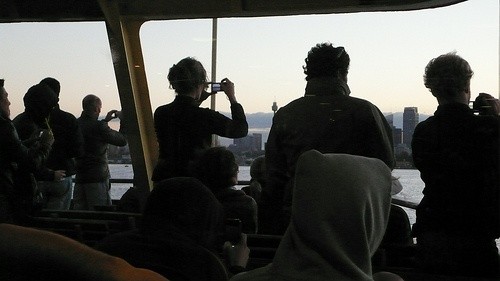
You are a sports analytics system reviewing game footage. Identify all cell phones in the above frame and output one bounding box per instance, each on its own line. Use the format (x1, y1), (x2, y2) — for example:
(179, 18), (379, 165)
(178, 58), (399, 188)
(224, 219), (242, 246)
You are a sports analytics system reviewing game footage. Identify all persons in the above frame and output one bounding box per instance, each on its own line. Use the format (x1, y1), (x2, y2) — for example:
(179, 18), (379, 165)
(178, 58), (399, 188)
(410, 51), (500, 281)
(263, 43), (395, 235)
(151, 58), (248, 188)
(0, 75), (404, 281)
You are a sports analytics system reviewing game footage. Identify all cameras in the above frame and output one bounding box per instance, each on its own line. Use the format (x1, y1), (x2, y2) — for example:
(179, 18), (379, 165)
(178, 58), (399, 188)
(115, 111), (122, 117)
(468, 101), (478, 112)
(212, 83), (224, 91)
